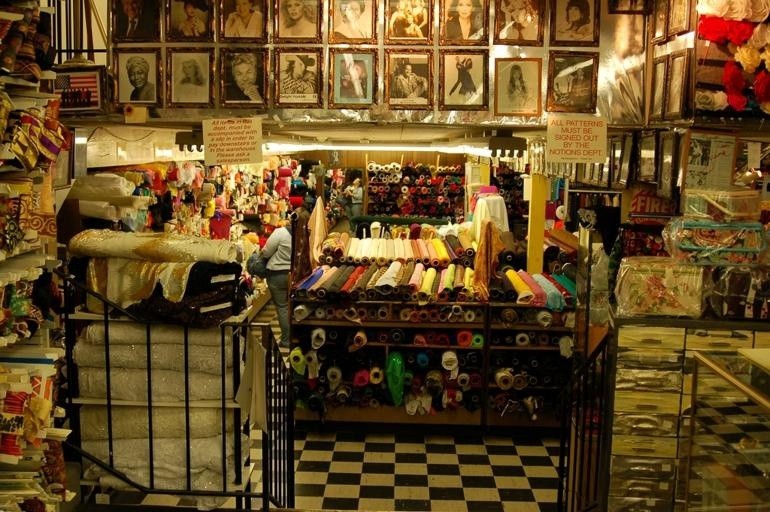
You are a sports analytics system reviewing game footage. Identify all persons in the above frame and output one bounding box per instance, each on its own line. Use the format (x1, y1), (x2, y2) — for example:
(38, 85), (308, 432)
(690, 135), (707, 165)
(125, 46), (590, 114)
(259, 202), (306, 347)
(108, 1), (592, 46)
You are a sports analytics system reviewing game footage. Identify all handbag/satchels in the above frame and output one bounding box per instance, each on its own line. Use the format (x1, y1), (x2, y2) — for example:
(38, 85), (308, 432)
(247, 253), (267, 279)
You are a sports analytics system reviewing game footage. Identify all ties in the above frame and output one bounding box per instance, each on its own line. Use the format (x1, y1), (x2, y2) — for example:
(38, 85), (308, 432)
(127, 19), (136, 36)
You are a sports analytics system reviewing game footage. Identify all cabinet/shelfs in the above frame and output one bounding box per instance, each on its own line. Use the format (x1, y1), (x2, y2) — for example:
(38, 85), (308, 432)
(284, 282), (613, 428)
(60, 258), (249, 512)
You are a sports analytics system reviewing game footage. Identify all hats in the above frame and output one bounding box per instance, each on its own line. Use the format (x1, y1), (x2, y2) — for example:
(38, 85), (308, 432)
(286, 55), (315, 66)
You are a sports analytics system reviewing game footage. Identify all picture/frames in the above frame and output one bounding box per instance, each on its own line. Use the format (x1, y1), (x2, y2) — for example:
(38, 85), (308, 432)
(546, 50), (600, 114)
(438, 48), (489, 111)
(549, 1), (600, 47)
(112, 47), (163, 109)
(383, 47), (434, 110)
(219, 47), (269, 110)
(166, 47), (217, 108)
(328, 47), (380, 110)
(439, 1), (489, 46)
(216, 1), (268, 44)
(51, 65), (107, 115)
(328, 2), (378, 45)
(493, 1), (546, 47)
(164, 2), (215, 43)
(52, 128), (74, 191)
(110, 1), (162, 43)
(273, 46), (323, 110)
(384, 2), (434, 45)
(581, 0), (691, 202)
(272, 1), (323, 43)
(494, 58), (542, 117)
